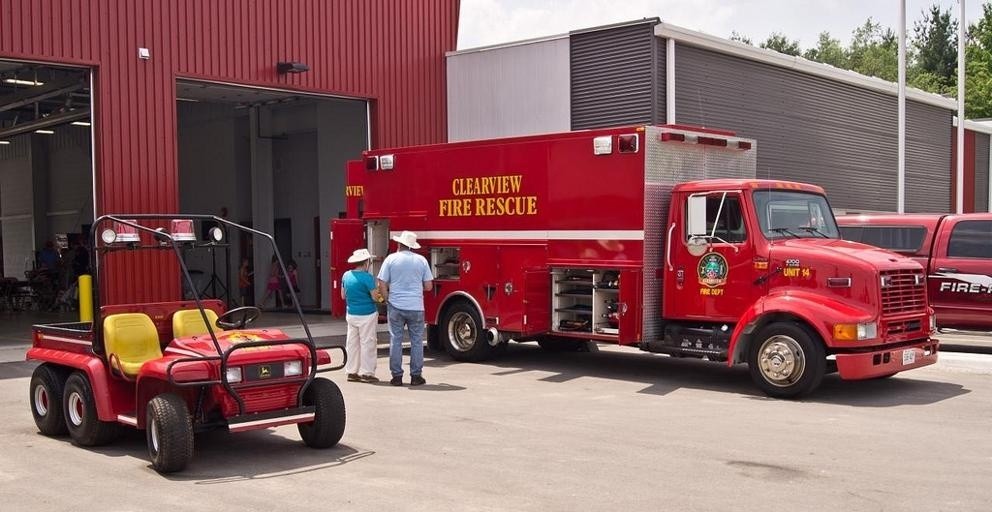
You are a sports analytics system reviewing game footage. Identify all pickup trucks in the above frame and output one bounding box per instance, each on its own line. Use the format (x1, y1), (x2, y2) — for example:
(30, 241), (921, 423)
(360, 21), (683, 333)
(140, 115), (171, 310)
(805, 211), (992, 340)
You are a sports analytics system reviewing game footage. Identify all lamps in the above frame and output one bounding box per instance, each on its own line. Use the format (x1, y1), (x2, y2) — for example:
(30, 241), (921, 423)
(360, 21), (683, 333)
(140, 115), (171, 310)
(278, 61), (310, 73)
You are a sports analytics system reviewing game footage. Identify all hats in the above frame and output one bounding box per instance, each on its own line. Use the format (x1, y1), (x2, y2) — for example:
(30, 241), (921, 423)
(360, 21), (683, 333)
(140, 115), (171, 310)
(391, 230), (422, 251)
(347, 248), (379, 264)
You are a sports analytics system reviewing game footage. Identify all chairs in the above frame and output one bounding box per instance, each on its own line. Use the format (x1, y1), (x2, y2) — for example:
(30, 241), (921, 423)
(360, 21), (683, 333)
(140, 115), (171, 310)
(171, 307), (226, 341)
(103, 314), (164, 376)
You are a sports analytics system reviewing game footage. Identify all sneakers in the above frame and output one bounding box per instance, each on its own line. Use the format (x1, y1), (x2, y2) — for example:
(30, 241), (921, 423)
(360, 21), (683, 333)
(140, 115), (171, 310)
(346, 373), (360, 382)
(390, 375), (403, 385)
(411, 374), (426, 385)
(361, 374), (379, 383)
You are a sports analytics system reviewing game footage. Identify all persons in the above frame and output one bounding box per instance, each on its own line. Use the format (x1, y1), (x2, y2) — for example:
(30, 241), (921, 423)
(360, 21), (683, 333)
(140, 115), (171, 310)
(282, 260), (298, 301)
(259, 254), (288, 309)
(340, 248), (381, 383)
(377, 231), (434, 386)
(239, 257), (253, 306)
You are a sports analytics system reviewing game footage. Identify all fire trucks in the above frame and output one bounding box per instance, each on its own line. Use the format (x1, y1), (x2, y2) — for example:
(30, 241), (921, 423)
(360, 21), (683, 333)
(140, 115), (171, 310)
(327, 116), (940, 403)
